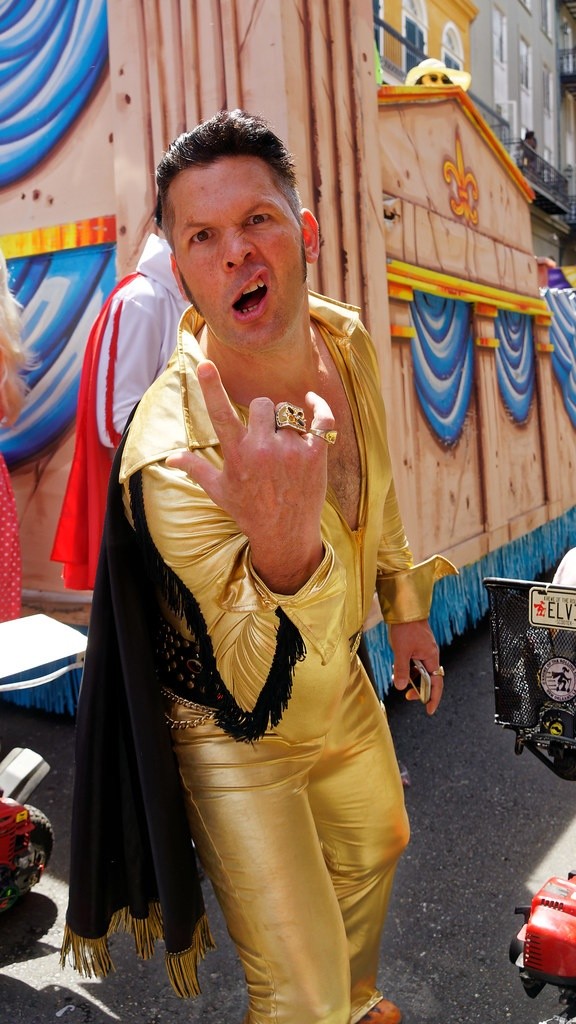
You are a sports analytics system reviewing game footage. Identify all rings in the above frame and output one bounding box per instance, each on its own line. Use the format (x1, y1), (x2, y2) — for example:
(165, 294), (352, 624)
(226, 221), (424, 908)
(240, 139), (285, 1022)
(430, 666), (444, 676)
(310, 428), (337, 444)
(276, 402), (307, 434)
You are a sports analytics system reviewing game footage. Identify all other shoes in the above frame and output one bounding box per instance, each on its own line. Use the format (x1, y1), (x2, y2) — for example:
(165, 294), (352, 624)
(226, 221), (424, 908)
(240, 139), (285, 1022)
(348, 986), (402, 1024)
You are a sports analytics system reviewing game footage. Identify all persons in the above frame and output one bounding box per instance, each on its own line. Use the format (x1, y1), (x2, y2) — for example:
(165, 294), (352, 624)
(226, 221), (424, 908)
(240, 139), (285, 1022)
(57, 110), (457, 1023)
(0, 250), (39, 621)
(53, 192), (190, 592)
